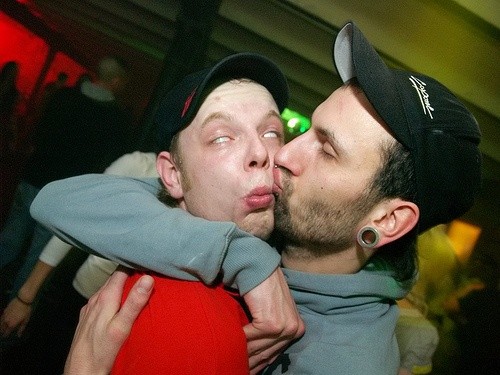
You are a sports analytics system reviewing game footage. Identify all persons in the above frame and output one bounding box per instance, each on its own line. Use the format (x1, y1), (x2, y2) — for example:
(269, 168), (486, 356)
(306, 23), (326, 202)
(0, 56), (146, 305)
(394, 228), (468, 374)
(0, 151), (160, 375)
(104, 52), (289, 374)
(27, 17), (482, 375)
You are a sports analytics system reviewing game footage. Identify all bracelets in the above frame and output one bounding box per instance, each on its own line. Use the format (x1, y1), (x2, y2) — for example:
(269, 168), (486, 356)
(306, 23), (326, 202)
(15, 294), (35, 306)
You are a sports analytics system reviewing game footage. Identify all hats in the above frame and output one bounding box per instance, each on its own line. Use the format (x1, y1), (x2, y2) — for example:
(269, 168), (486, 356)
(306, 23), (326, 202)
(331, 23), (485, 216)
(151, 50), (291, 154)
